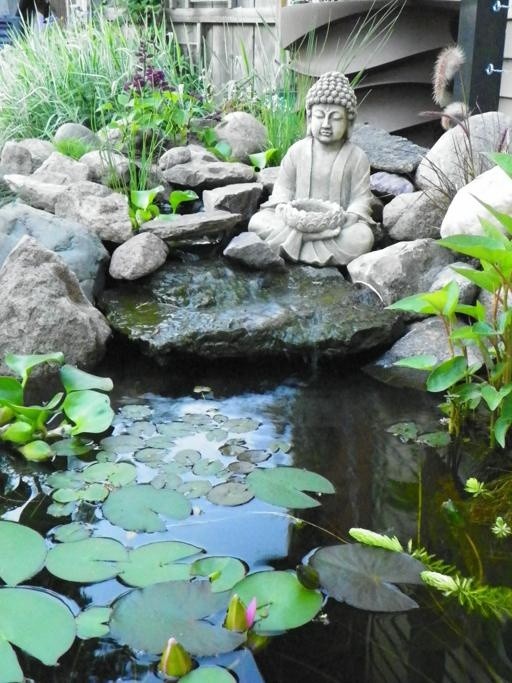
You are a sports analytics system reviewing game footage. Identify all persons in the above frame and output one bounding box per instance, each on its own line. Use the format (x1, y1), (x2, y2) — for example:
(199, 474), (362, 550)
(245, 70), (378, 268)
